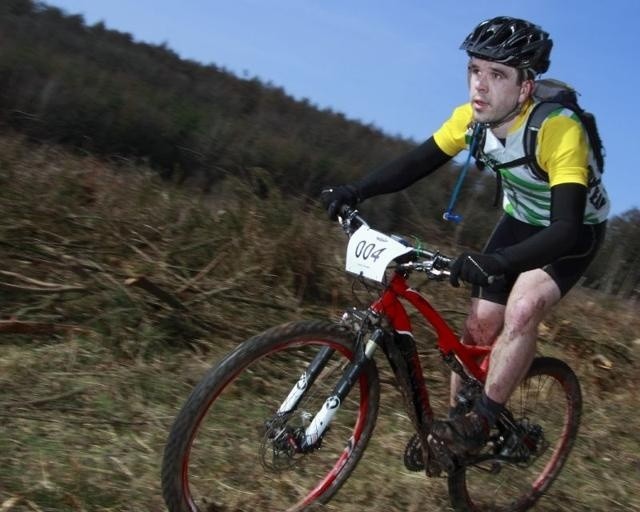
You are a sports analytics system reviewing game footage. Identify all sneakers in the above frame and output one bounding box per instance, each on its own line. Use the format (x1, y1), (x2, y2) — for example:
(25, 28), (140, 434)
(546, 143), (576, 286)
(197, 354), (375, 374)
(432, 412), (487, 460)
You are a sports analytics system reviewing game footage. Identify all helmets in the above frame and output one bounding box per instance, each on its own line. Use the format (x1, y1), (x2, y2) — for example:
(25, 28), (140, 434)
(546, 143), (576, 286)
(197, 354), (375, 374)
(460, 16), (552, 80)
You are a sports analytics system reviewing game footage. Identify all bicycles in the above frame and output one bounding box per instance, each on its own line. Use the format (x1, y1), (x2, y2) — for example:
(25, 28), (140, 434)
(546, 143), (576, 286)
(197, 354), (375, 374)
(162, 185), (583, 512)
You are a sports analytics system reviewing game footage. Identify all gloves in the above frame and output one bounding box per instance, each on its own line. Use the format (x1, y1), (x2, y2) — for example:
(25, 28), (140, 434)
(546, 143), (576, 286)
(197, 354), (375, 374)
(450, 253), (504, 287)
(320, 184), (357, 221)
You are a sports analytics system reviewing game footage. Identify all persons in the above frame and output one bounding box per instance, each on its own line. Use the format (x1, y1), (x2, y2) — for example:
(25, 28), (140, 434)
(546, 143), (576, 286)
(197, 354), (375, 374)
(321, 17), (612, 455)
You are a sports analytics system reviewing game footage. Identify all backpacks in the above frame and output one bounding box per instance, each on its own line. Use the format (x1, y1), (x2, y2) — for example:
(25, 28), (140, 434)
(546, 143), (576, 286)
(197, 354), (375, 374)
(530, 78), (603, 187)
